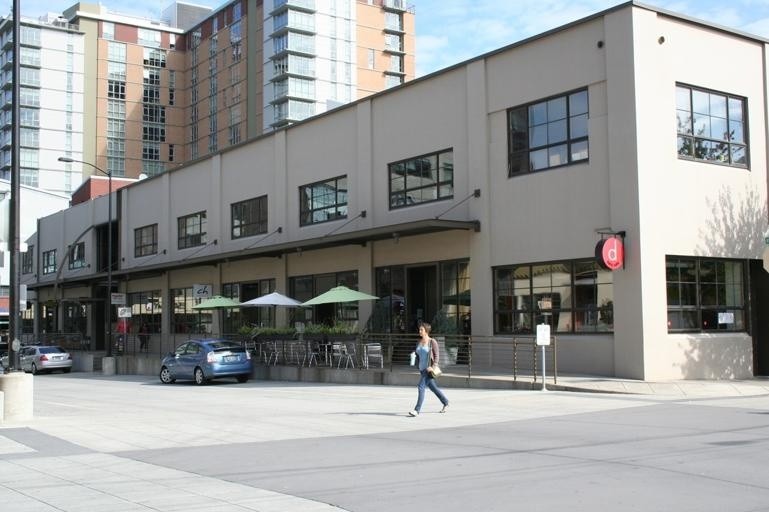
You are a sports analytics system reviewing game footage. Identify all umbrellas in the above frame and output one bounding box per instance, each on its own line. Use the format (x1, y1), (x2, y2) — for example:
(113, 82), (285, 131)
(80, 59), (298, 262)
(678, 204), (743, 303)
(299, 286), (382, 322)
(237, 292), (313, 329)
(442, 289), (470, 307)
(191, 296), (244, 339)
(378, 294), (405, 307)
(340, 303), (358, 311)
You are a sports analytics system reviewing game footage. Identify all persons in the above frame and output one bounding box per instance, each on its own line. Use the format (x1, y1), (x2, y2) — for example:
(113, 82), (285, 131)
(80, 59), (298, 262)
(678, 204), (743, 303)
(115, 317), (134, 356)
(462, 309), (471, 356)
(137, 322), (152, 354)
(408, 323), (451, 417)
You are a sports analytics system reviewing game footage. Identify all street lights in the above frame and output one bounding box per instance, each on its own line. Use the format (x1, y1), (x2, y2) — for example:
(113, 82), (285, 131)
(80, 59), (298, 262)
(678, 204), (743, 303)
(56, 153), (118, 379)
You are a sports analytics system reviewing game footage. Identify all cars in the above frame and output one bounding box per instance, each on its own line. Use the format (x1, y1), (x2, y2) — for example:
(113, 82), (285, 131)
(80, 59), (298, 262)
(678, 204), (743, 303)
(158, 338), (256, 387)
(0, 342), (77, 378)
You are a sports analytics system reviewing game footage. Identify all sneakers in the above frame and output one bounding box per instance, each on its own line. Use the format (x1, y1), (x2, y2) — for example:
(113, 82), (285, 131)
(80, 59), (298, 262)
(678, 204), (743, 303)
(440, 403), (450, 413)
(409, 410), (419, 416)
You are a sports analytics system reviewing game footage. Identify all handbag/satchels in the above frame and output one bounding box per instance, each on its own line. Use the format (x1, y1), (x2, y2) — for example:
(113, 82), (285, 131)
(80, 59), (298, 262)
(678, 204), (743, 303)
(430, 365), (442, 379)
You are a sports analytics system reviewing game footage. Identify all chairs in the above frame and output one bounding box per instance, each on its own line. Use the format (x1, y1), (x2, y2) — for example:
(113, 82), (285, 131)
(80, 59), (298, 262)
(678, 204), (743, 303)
(244, 339), (384, 370)
